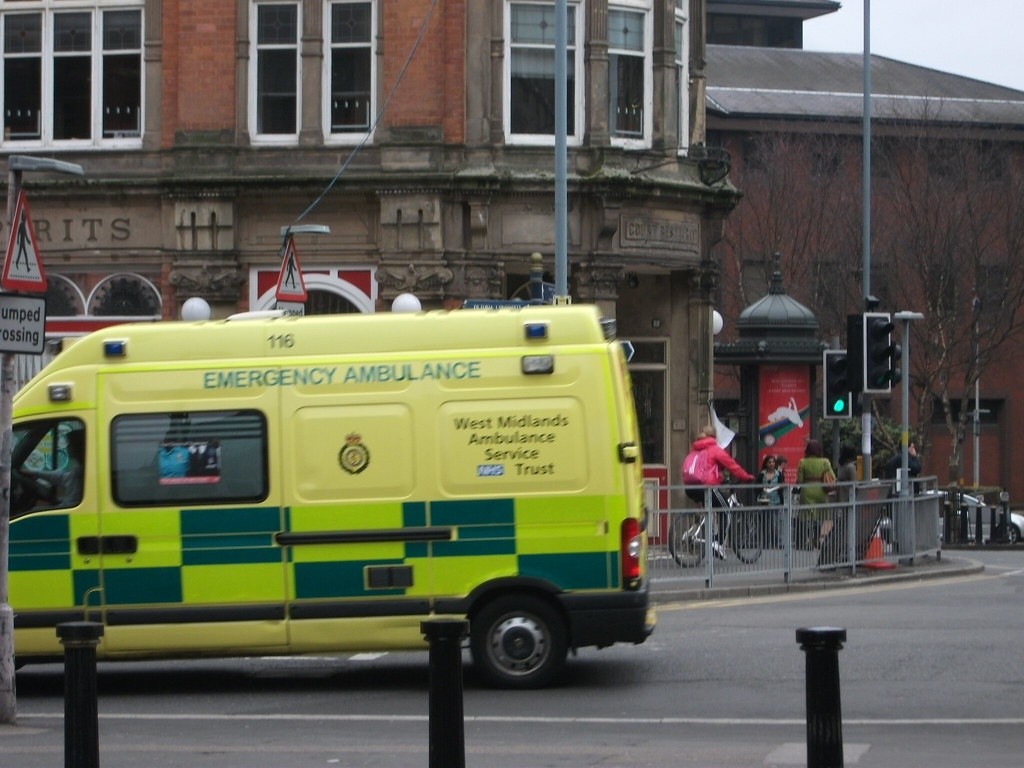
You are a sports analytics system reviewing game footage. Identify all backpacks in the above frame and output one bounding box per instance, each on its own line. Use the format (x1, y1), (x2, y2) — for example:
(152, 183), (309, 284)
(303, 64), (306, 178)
(682, 449), (708, 484)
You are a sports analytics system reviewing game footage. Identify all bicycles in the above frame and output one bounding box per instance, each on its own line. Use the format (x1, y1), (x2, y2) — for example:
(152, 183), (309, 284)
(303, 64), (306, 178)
(668, 478), (764, 569)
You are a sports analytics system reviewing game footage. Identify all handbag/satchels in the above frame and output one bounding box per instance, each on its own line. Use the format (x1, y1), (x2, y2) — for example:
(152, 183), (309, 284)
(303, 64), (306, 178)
(823, 471), (834, 488)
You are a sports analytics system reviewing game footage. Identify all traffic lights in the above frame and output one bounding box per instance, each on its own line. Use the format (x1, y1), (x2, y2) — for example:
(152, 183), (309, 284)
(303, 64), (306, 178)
(864, 313), (894, 393)
(823, 351), (852, 419)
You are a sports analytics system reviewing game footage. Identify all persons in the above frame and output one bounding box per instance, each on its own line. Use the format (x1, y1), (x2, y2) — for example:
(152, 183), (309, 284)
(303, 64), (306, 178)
(775, 456), (787, 474)
(838, 445), (860, 511)
(884, 438), (922, 553)
(681, 424), (756, 560)
(795, 440), (837, 550)
(19, 428), (86, 509)
(756, 454), (788, 546)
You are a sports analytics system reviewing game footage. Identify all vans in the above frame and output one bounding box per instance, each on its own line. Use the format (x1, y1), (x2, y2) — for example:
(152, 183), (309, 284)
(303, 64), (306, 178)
(10, 303), (659, 691)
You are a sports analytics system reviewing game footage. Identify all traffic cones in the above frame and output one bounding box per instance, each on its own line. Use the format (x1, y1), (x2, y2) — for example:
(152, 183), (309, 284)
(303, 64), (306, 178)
(862, 525), (897, 569)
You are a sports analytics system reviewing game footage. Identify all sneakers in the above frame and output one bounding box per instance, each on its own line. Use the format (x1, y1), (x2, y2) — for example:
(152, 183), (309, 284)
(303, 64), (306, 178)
(713, 541), (729, 560)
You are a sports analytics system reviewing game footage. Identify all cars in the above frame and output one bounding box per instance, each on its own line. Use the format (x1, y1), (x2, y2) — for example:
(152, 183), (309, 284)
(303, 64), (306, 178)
(879, 490), (1024, 545)
